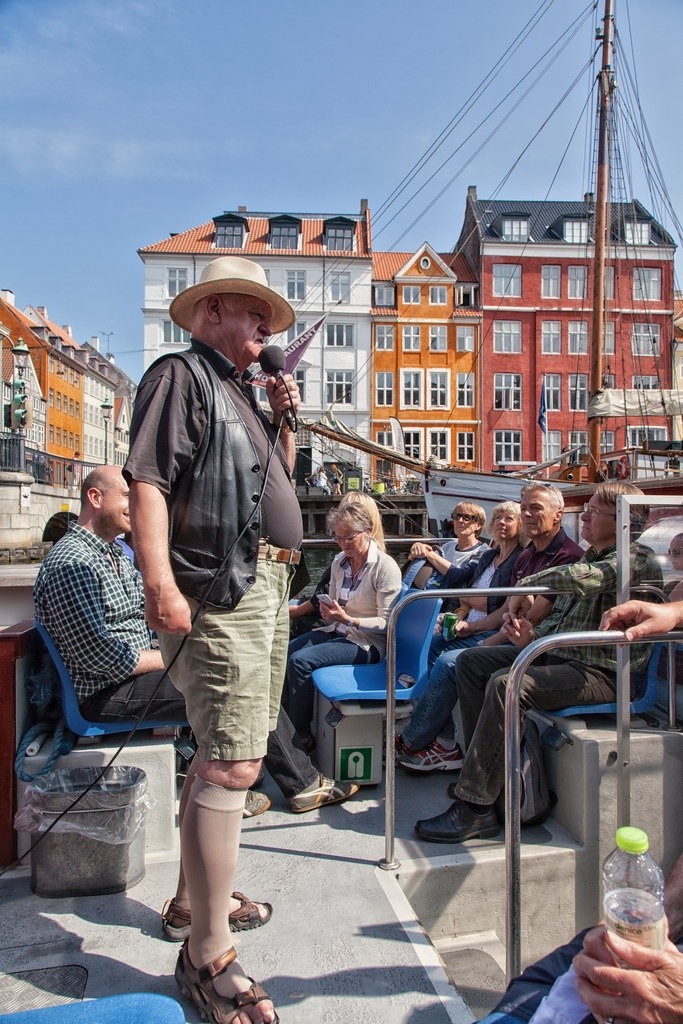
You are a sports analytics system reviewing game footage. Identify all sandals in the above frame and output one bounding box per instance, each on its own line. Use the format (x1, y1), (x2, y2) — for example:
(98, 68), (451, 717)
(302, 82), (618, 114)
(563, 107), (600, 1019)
(161, 892), (273, 942)
(175, 936), (279, 1024)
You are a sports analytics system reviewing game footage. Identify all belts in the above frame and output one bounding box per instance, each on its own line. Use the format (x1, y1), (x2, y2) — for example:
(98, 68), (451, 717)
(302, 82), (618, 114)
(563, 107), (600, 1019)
(257, 546), (302, 566)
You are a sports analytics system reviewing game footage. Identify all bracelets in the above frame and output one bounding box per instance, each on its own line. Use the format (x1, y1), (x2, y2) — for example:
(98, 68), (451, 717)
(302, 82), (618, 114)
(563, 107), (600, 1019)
(454, 608), (467, 615)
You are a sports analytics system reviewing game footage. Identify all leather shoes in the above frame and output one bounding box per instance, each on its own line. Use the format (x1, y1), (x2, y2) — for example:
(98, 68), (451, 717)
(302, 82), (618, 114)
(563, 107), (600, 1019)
(448, 782), (457, 797)
(415, 799), (499, 843)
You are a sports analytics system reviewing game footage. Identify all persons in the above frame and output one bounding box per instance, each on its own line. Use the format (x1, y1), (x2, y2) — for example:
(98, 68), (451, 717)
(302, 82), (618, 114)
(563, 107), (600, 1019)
(32, 442), (53, 485)
(472, 852), (683, 1024)
(657, 532), (683, 683)
(385, 469), (395, 495)
(33, 464), (404, 817)
(70, 451), (81, 490)
(598, 600), (683, 647)
(413, 480), (663, 843)
(407, 501), (531, 623)
(123, 256), (302, 1024)
(382, 482), (586, 771)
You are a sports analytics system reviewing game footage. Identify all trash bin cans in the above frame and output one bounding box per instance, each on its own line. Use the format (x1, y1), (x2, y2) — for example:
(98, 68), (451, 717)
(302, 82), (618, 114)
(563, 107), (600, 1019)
(26, 765), (148, 898)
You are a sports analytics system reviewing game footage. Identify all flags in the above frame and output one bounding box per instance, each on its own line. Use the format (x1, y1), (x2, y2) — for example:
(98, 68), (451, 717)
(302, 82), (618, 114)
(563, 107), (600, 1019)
(538, 381), (547, 433)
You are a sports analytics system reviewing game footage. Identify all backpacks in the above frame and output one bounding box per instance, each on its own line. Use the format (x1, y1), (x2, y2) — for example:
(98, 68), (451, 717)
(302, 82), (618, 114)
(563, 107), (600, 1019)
(400, 545), (450, 600)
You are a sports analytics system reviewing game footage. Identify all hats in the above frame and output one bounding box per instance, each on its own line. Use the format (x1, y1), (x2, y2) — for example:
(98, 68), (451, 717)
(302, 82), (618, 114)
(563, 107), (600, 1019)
(169, 256), (296, 335)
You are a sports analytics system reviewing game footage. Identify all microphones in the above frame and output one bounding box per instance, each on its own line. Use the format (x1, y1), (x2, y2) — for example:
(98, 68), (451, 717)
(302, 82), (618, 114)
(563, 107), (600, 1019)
(259, 345), (296, 434)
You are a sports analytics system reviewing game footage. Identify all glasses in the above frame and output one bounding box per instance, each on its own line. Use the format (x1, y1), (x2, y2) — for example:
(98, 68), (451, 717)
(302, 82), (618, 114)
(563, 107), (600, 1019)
(584, 503), (614, 517)
(543, 484), (560, 503)
(334, 533), (358, 543)
(450, 513), (476, 521)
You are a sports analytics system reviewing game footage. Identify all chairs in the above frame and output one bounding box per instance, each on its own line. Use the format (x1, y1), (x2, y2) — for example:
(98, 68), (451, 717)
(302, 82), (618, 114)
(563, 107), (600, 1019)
(312, 540), (667, 714)
(37, 623), (195, 740)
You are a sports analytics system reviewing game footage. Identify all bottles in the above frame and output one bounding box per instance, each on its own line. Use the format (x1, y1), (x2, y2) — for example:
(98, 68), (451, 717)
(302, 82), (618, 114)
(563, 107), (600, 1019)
(602, 826), (668, 969)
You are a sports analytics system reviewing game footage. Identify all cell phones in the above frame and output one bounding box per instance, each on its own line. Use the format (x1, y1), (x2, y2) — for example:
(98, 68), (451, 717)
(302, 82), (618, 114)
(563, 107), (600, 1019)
(317, 594), (335, 610)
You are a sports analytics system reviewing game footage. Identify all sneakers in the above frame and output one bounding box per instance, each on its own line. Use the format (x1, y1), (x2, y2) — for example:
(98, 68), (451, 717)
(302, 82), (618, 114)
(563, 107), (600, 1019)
(381, 734), (465, 770)
(290, 773), (359, 812)
(242, 790), (272, 817)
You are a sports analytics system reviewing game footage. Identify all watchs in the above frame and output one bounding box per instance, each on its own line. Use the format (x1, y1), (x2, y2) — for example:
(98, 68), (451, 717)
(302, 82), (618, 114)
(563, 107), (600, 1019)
(477, 640), (484, 646)
(347, 618), (354, 627)
